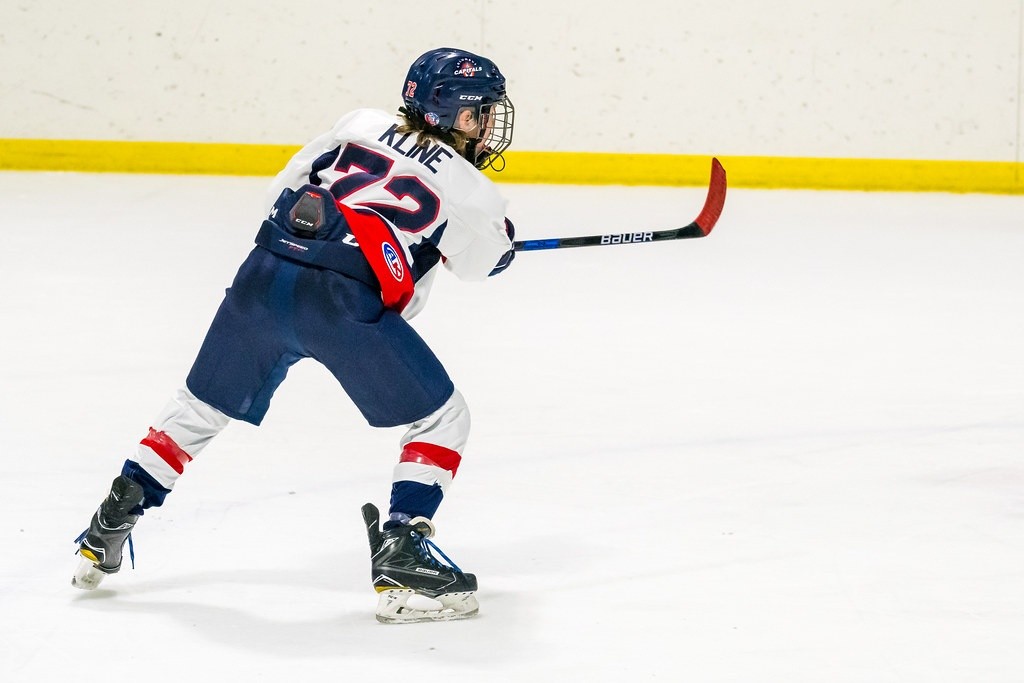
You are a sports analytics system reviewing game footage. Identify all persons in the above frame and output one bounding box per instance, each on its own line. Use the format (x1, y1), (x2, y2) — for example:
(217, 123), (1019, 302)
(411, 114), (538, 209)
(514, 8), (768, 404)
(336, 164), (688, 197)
(70, 46), (516, 624)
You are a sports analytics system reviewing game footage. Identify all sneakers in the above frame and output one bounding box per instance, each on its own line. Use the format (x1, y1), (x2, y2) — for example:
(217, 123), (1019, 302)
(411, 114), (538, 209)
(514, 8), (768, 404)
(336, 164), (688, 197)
(361, 502), (480, 624)
(72, 476), (144, 590)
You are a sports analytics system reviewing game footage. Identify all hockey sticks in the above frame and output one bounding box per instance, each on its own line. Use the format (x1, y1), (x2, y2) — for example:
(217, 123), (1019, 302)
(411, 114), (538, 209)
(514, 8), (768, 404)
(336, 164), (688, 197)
(513, 155), (727, 252)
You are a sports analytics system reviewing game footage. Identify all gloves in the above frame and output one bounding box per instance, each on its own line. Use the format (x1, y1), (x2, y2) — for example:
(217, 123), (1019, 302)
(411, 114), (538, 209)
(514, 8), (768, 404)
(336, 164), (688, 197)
(488, 216), (515, 276)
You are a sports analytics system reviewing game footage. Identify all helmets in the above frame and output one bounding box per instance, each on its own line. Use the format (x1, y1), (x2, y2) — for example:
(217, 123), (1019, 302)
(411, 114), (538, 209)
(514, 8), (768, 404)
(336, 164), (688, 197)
(401, 48), (514, 170)
